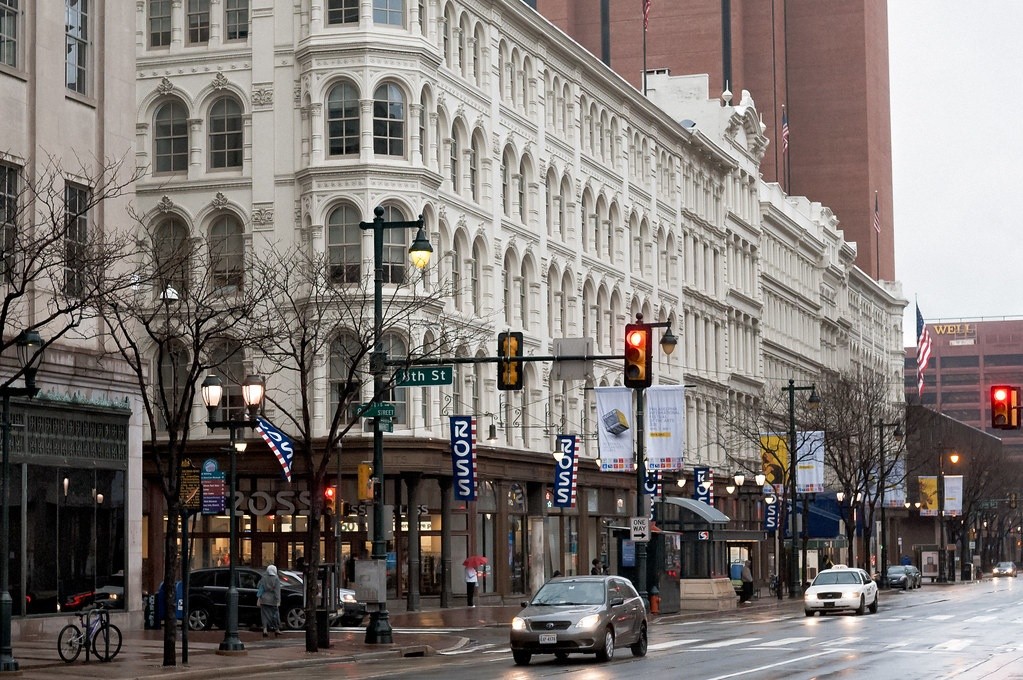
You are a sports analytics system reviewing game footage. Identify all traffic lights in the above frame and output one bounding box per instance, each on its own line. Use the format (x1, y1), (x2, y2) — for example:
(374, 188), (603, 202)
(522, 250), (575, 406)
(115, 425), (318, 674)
(623, 324), (652, 389)
(355, 462), (374, 503)
(990, 384), (1012, 430)
(325, 487), (336, 516)
(341, 499), (350, 518)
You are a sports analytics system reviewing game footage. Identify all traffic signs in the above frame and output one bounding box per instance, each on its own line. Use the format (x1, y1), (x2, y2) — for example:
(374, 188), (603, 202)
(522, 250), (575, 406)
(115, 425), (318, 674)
(629, 516), (651, 542)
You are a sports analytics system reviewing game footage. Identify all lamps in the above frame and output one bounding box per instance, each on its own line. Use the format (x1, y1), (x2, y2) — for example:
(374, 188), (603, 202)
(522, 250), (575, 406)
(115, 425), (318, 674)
(487, 414), (498, 443)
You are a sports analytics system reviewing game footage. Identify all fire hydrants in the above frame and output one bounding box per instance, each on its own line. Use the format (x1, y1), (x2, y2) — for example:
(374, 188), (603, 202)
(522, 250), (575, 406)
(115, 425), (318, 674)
(649, 586), (661, 615)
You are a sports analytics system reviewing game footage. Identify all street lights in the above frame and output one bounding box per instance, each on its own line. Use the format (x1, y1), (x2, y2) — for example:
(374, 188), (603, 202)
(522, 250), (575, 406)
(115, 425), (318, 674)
(782, 380), (820, 600)
(63, 477), (104, 593)
(634, 312), (678, 615)
(202, 374), (264, 657)
(874, 417), (905, 590)
(360, 205), (434, 643)
(0, 329), (45, 671)
(932, 442), (960, 582)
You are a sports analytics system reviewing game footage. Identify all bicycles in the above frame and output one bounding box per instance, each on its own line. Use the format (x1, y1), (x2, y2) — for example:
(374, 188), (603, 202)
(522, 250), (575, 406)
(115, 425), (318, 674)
(57, 600), (122, 664)
(769, 574), (778, 597)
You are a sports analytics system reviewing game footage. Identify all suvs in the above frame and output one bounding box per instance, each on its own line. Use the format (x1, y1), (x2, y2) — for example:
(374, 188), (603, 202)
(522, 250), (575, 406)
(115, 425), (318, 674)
(510, 575), (648, 664)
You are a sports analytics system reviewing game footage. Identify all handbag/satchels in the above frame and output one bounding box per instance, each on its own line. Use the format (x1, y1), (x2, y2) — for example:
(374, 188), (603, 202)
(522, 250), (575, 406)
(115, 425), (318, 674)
(256, 577), (264, 597)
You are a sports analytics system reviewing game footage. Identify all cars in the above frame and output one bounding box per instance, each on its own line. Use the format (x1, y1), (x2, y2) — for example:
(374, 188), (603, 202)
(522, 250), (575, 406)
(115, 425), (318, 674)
(185, 566), (356, 629)
(887, 565), (922, 590)
(66, 571), (125, 610)
(804, 565), (880, 617)
(993, 561), (1017, 578)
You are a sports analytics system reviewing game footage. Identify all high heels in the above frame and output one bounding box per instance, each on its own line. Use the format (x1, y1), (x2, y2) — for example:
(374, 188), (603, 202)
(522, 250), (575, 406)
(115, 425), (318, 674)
(263, 633), (268, 637)
(275, 632), (284, 637)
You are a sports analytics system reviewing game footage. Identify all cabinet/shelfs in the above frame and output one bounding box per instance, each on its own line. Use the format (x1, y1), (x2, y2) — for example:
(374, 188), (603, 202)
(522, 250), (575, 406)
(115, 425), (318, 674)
(421, 556), (432, 594)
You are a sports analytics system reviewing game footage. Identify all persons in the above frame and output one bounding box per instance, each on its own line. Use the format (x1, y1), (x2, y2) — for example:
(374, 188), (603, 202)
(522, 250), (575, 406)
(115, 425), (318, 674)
(591, 559), (609, 575)
(741, 559), (753, 604)
(901, 554), (911, 565)
(822, 555), (834, 569)
(255, 564), (286, 638)
(464, 566), (480, 608)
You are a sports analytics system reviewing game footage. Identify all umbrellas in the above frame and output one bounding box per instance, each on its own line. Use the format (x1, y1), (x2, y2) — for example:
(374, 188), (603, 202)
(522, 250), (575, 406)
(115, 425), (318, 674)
(462, 554), (488, 568)
